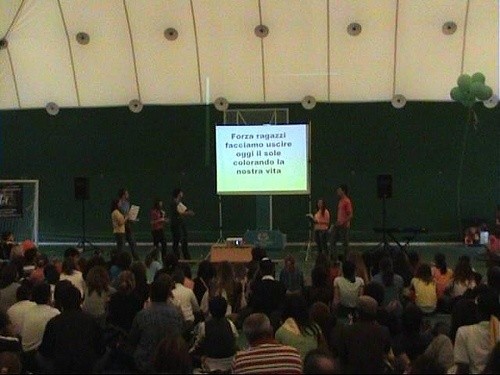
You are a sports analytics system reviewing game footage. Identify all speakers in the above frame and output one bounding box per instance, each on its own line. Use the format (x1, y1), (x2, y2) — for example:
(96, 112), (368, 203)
(377, 174), (392, 198)
(74, 177), (90, 201)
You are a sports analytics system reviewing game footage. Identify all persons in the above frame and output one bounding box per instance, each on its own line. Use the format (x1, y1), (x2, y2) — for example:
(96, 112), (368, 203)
(119, 189), (141, 261)
(111, 197), (131, 253)
(312, 199), (330, 255)
(0, 215), (500, 375)
(170, 189), (193, 260)
(149, 199), (167, 259)
(329, 185), (352, 258)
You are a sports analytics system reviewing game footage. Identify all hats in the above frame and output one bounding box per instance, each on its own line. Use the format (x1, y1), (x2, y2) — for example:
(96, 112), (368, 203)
(261, 258), (273, 270)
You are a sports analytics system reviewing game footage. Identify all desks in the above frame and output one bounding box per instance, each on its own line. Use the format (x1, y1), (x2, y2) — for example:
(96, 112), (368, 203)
(374, 227), (429, 251)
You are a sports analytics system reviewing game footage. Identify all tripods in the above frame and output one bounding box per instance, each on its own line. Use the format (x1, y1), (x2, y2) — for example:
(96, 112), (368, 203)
(76, 200), (95, 254)
(373, 198), (395, 253)
